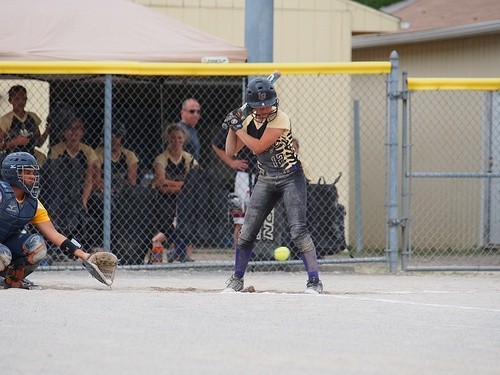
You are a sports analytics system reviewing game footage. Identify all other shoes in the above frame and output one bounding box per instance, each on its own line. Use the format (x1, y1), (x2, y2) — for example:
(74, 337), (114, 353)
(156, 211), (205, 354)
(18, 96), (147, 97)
(221, 275), (244, 293)
(304, 276), (324, 295)
(0, 274), (42, 291)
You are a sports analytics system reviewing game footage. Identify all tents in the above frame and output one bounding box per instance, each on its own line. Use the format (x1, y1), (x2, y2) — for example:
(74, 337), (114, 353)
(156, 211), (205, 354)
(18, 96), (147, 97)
(0, 0), (246, 246)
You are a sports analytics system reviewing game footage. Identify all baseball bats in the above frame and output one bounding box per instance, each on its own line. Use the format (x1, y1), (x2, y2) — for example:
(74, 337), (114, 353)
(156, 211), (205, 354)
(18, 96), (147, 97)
(221, 71), (282, 129)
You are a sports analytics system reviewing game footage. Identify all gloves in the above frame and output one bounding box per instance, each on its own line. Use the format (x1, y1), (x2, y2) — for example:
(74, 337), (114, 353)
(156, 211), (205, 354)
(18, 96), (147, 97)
(224, 109), (245, 132)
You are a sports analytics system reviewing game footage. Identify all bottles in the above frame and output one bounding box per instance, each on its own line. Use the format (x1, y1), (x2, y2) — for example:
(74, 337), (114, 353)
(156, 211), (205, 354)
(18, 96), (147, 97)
(19, 125), (28, 136)
(144, 171), (154, 186)
(153, 240), (163, 264)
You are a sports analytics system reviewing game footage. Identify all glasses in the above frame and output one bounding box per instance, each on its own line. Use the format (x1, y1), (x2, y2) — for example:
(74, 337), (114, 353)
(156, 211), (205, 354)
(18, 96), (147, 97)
(183, 109), (201, 114)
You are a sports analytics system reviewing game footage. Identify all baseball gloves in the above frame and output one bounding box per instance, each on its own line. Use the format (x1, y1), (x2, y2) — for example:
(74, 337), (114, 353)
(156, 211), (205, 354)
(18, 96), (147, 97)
(84, 252), (118, 287)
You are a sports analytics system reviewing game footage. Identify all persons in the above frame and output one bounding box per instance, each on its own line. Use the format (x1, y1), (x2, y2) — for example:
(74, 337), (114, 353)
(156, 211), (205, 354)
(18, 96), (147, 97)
(225, 77), (323, 294)
(177, 98), (200, 260)
(0, 152), (118, 289)
(230, 136), (259, 243)
(293, 138), (298, 154)
(0, 85), (50, 158)
(95, 128), (139, 191)
(154, 123), (201, 194)
(50, 118), (98, 212)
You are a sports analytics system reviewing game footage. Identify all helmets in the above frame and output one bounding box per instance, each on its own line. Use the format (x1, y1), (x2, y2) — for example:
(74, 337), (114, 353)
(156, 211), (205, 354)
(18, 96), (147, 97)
(245, 76), (278, 109)
(1, 151), (41, 188)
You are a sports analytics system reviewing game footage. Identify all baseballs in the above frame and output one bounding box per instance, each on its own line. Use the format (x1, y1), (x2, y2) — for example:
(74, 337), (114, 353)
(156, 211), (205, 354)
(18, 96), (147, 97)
(274, 246), (290, 261)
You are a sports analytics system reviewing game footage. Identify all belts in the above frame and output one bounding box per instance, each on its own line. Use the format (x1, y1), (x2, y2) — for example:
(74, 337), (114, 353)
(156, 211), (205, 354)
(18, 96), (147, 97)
(259, 161), (302, 177)
